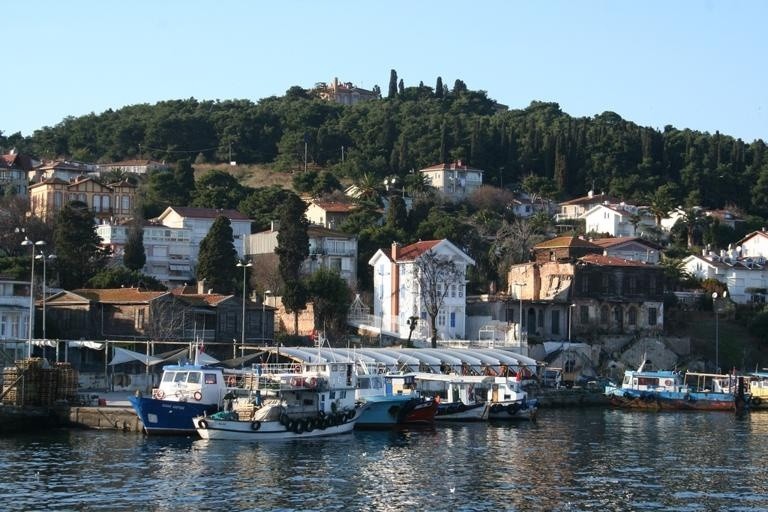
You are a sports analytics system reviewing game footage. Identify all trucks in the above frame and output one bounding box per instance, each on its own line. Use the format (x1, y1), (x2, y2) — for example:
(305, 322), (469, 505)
(540, 367), (575, 390)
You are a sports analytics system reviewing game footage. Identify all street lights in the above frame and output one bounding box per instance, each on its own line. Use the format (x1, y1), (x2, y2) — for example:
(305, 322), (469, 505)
(235, 259), (254, 370)
(568, 304), (576, 376)
(262, 290), (271, 347)
(514, 283), (527, 354)
(20, 236), (50, 369)
(712, 290), (727, 368)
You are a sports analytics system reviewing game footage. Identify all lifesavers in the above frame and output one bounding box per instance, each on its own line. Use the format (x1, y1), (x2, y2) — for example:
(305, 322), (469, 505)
(290, 378), (296, 386)
(194, 392), (202, 400)
(665, 380), (670, 386)
(154, 390), (164, 399)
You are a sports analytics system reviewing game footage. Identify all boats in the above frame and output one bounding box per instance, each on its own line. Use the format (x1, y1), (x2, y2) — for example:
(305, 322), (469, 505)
(601, 352), (768, 411)
(190, 358), (372, 439)
(0, 405), (51, 435)
(127, 335), (539, 439)
